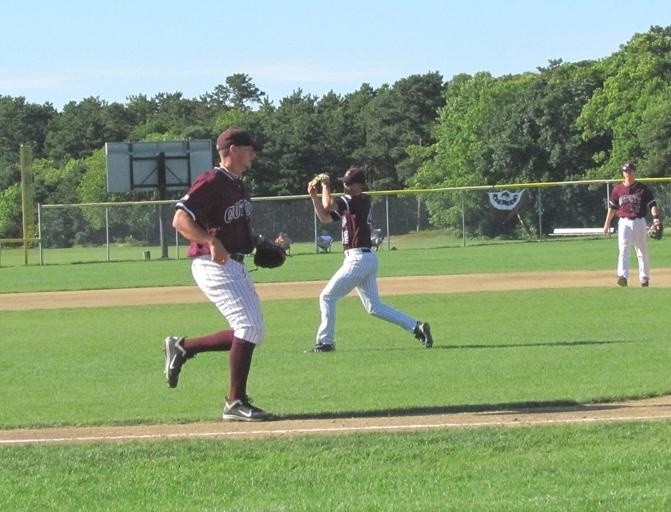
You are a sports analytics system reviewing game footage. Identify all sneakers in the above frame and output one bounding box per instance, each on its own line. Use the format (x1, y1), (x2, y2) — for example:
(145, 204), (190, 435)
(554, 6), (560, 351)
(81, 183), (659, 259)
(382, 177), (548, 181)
(163, 336), (187, 388)
(303, 342), (336, 354)
(639, 278), (649, 287)
(617, 276), (627, 287)
(222, 395), (274, 421)
(413, 321), (433, 349)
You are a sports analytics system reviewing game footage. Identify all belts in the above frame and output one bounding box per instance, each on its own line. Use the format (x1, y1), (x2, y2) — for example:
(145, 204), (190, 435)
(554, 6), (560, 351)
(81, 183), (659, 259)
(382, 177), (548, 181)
(345, 249), (371, 256)
(620, 216), (643, 220)
(230, 254), (244, 262)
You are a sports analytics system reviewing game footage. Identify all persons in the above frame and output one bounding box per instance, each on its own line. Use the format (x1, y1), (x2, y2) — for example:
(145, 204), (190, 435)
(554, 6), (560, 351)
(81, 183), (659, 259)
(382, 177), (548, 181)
(303, 169), (433, 353)
(602, 163), (663, 286)
(275, 232), (290, 249)
(371, 228), (383, 250)
(318, 229), (332, 252)
(166, 128), (273, 422)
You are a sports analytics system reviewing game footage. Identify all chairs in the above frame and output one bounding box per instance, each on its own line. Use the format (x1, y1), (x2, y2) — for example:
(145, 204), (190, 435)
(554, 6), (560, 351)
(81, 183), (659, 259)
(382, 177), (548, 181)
(317, 235), (334, 253)
(280, 238), (292, 256)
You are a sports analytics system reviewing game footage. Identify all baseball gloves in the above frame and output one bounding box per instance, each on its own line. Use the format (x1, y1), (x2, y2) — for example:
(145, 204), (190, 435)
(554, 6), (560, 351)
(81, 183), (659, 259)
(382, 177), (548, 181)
(254, 240), (285, 268)
(647, 223), (662, 240)
(311, 173), (329, 186)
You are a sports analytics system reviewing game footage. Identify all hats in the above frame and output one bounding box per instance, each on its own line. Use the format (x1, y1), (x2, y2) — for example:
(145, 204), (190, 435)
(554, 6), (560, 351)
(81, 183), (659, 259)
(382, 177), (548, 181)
(343, 169), (367, 184)
(216, 128), (262, 152)
(622, 163), (637, 172)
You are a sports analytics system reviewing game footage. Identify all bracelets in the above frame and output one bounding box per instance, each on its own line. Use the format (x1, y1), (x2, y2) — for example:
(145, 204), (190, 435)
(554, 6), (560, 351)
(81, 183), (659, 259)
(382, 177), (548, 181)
(652, 215), (660, 219)
